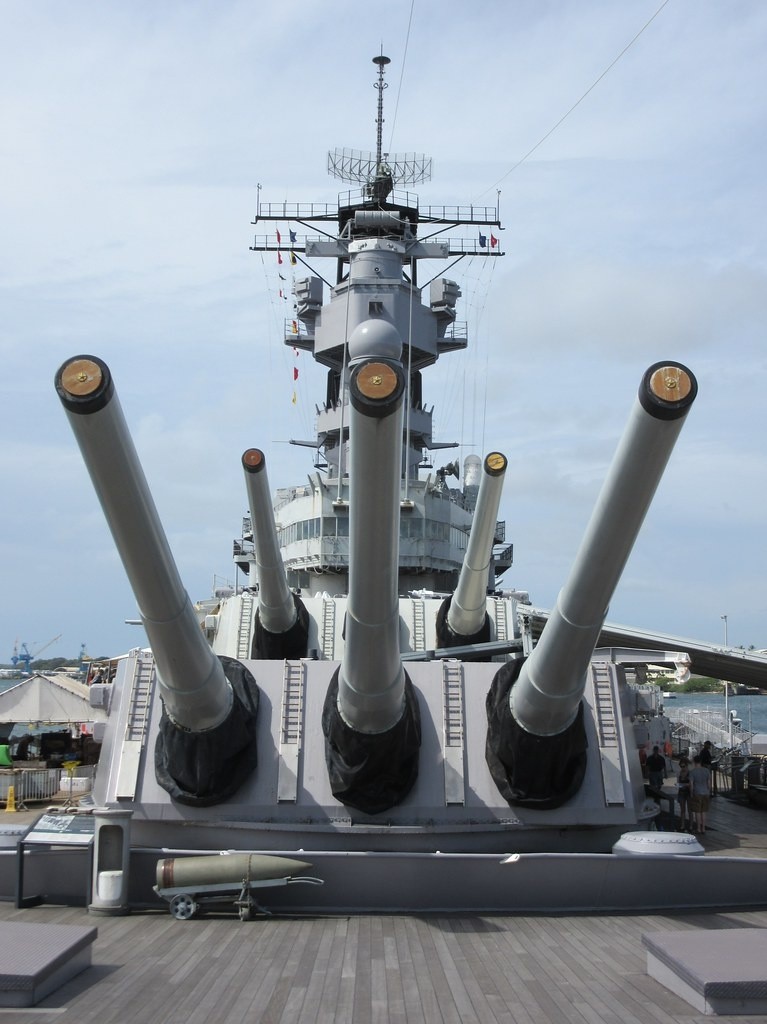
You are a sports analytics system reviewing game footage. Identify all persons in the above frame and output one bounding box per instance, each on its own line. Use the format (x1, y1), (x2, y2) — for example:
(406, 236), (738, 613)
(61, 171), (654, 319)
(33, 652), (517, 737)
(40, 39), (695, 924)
(90, 668), (116, 683)
(690, 756), (711, 834)
(17, 736), (34, 760)
(675, 758), (694, 830)
(698, 741), (712, 770)
(646, 745), (667, 789)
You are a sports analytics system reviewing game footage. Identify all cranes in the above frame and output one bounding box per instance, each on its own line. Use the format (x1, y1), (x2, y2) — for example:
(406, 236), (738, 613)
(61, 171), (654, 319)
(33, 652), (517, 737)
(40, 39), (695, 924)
(19, 633), (63, 671)
(11, 638), (19, 670)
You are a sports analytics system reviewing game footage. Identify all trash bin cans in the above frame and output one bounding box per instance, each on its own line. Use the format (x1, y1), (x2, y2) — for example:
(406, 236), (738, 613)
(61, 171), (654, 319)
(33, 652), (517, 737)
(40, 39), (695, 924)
(87, 810), (135, 916)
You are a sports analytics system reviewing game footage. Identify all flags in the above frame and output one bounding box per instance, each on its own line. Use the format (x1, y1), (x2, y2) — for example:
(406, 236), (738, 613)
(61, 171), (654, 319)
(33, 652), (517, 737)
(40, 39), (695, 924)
(277, 232), (280, 242)
(292, 305), (298, 380)
(277, 251), (282, 264)
(491, 235), (498, 248)
(289, 230), (297, 242)
(279, 275), (286, 298)
(292, 392), (297, 404)
(479, 232), (486, 247)
(290, 254), (297, 265)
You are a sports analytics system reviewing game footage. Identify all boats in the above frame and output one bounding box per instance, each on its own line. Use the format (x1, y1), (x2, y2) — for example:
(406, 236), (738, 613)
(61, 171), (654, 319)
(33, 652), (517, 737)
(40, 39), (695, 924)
(663, 691), (677, 698)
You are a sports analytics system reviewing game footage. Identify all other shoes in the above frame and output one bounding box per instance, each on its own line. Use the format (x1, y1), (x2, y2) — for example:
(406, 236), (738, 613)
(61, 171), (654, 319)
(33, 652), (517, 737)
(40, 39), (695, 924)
(680, 826), (686, 830)
(702, 829), (706, 833)
(697, 828), (701, 833)
(689, 826), (693, 830)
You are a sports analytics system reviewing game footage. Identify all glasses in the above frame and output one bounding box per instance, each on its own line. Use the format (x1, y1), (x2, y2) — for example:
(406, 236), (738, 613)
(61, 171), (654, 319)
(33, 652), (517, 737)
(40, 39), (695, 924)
(679, 765), (686, 768)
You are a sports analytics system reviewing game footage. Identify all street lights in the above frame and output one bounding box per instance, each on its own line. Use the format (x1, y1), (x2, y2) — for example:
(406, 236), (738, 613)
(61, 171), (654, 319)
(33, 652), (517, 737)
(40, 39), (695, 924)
(720, 615), (728, 730)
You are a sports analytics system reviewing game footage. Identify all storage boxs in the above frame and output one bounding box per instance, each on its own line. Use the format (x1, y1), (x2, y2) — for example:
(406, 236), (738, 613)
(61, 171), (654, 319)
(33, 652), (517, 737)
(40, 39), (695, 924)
(60, 777), (91, 792)
(47, 753), (81, 767)
